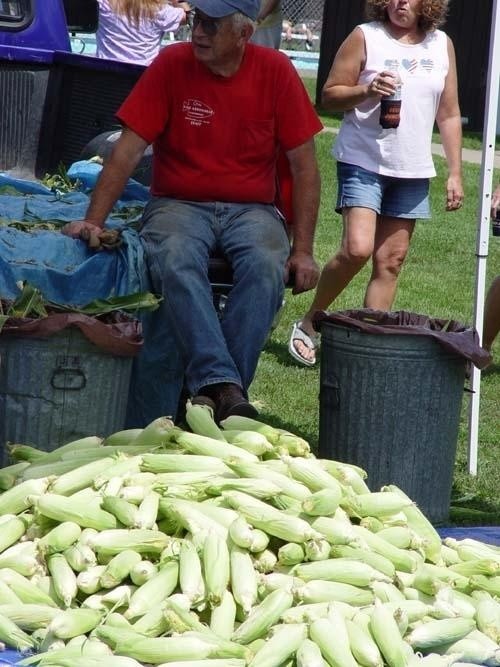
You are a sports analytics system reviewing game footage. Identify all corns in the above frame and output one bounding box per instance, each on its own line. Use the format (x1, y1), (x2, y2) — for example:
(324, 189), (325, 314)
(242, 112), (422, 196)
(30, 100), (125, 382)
(0, 403), (500, 667)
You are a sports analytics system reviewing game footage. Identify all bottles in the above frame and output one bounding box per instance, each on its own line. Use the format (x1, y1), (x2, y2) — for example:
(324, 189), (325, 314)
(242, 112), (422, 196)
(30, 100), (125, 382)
(379, 60), (402, 130)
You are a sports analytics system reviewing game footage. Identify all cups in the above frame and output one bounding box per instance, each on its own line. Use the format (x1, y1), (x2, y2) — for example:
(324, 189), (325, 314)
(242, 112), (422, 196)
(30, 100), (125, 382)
(492, 206), (500, 236)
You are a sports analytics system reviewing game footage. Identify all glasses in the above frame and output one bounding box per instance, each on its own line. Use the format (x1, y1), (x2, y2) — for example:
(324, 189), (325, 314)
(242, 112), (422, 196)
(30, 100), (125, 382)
(185, 8), (219, 36)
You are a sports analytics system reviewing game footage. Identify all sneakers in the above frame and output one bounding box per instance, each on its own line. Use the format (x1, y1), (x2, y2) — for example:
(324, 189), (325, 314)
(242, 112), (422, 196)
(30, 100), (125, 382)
(191, 393), (216, 410)
(215, 385), (258, 424)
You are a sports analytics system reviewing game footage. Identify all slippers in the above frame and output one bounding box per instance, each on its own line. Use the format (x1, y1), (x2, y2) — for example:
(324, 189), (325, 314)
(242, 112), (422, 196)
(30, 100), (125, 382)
(287, 320), (318, 366)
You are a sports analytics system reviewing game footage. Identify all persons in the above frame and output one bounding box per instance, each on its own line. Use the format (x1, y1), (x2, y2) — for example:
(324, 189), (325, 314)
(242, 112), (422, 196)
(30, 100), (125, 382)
(481, 177), (500, 359)
(245, 0), (284, 54)
(59, 0), (327, 428)
(285, 0), (469, 369)
(89, 0), (192, 71)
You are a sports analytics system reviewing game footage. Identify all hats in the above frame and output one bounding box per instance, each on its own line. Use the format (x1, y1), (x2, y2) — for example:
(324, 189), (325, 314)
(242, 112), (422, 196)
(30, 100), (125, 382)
(178, 1), (261, 24)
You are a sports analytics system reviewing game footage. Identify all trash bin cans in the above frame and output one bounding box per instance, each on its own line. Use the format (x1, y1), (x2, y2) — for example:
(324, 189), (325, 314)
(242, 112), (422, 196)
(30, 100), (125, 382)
(0, 310), (144, 469)
(311, 308), (493, 528)
